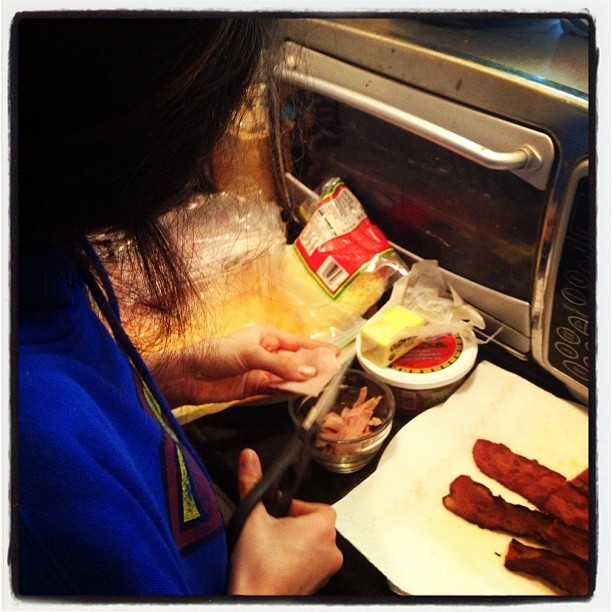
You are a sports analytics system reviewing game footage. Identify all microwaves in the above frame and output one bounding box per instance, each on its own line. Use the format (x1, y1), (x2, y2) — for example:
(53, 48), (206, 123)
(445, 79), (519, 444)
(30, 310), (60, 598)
(265, 12), (589, 408)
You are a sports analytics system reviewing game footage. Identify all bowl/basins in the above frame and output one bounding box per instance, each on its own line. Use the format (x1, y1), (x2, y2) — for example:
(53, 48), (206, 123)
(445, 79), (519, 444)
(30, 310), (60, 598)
(289, 368), (395, 476)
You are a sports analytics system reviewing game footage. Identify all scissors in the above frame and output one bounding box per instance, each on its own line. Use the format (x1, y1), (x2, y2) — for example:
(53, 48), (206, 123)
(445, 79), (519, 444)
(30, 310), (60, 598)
(224, 353), (356, 543)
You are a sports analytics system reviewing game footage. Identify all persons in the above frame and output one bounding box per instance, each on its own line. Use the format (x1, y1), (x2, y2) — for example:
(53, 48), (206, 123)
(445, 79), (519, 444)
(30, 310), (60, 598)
(18, 17), (343, 597)
